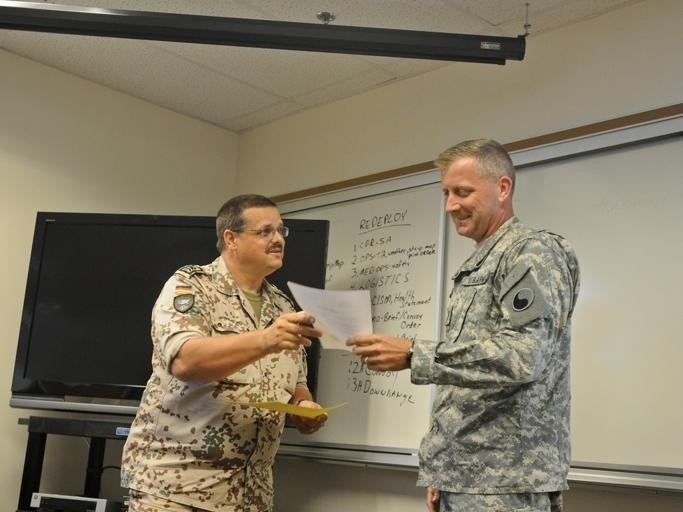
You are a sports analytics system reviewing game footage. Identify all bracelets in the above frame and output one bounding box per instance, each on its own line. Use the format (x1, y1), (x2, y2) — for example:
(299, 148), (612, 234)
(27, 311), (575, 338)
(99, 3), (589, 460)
(406, 338), (416, 369)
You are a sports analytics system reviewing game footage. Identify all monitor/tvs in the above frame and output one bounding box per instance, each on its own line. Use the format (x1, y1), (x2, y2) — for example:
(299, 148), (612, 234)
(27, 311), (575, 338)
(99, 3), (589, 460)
(10, 212), (330, 414)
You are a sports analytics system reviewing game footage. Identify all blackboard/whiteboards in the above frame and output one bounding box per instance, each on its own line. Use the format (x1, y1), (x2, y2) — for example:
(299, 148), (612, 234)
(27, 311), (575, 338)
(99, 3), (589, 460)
(439, 131), (683, 476)
(279, 177), (444, 448)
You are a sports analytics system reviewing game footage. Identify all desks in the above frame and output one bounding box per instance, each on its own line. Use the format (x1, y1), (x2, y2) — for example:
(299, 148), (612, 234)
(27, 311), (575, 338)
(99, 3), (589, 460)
(9, 418), (130, 511)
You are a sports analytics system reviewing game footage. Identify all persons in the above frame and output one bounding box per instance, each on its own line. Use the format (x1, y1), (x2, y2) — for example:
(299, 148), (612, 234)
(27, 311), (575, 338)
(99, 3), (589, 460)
(345, 137), (583, 512)
(119, 193), (329, 512)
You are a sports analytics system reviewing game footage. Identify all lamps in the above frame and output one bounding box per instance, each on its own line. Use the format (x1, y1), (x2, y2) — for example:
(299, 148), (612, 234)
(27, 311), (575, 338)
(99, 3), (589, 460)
(1, 0), (525, 69)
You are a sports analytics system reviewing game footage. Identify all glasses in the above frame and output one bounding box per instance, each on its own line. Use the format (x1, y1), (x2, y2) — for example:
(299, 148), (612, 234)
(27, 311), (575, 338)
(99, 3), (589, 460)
(236, 226), (289, 240)
